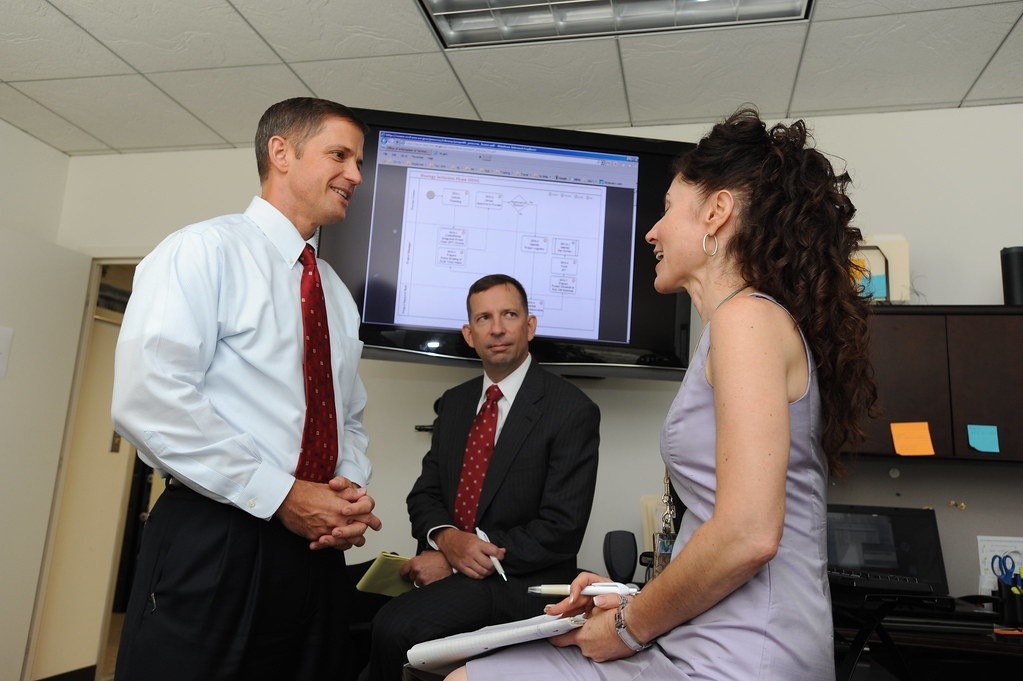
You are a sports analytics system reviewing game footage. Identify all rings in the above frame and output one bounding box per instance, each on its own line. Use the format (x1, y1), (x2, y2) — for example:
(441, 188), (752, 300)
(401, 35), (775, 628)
(414, 581), (419, 588)
(619, 594), (628, 605)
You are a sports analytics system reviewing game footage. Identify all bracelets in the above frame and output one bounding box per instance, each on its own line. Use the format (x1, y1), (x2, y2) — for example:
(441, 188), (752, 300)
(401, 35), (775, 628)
(615, 603), (655, 651)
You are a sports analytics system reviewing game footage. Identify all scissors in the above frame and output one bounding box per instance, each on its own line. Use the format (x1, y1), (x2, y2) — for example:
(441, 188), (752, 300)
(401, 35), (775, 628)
(991, 554), (1015, 585)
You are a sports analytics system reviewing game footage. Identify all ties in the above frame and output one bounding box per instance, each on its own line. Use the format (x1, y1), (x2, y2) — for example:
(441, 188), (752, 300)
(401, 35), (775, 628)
(452, 385), (504, 533)
(294, 243), (339, 485)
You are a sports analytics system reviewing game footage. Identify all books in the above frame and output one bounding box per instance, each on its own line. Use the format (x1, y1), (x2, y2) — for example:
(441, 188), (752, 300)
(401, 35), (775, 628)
(407, 614), (587, 668)
(356, 551), (415, 597)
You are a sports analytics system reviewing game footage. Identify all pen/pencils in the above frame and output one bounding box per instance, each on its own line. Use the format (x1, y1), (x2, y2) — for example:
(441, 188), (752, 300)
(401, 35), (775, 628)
(475, 526), (509, 582)
(528, 582), (638, 595)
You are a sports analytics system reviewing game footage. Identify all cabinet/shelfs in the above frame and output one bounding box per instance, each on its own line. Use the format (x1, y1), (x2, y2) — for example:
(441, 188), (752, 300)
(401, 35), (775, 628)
(836, 304), (1023, 461)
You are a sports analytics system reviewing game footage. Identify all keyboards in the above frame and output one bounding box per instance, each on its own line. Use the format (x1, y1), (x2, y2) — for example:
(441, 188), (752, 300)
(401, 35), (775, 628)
(827, 566), (933, 593)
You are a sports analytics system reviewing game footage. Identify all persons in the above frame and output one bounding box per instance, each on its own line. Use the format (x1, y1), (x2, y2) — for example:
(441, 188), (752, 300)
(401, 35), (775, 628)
(345, 274), (600, 681)
(110, 96), (381, 681)
(444, 107), (885, 681)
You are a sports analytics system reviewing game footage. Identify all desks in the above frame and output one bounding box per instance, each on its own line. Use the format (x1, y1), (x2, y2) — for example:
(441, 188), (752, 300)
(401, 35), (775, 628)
(833, 616), (1023, 681)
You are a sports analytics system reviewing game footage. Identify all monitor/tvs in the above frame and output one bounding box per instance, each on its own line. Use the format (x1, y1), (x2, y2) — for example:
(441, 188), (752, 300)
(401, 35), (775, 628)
(315, 107), (691, 381)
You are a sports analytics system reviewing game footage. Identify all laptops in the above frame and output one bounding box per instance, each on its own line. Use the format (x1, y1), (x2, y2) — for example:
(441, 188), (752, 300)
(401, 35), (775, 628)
(826, 504), (1001, 626)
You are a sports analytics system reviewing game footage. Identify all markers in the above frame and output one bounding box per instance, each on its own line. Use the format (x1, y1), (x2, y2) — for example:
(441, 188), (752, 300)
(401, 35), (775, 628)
(1009, 566), (1023, 594)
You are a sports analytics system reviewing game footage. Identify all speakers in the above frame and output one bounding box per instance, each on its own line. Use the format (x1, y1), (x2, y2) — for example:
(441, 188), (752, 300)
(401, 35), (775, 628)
(603, 530), (638, 584)
